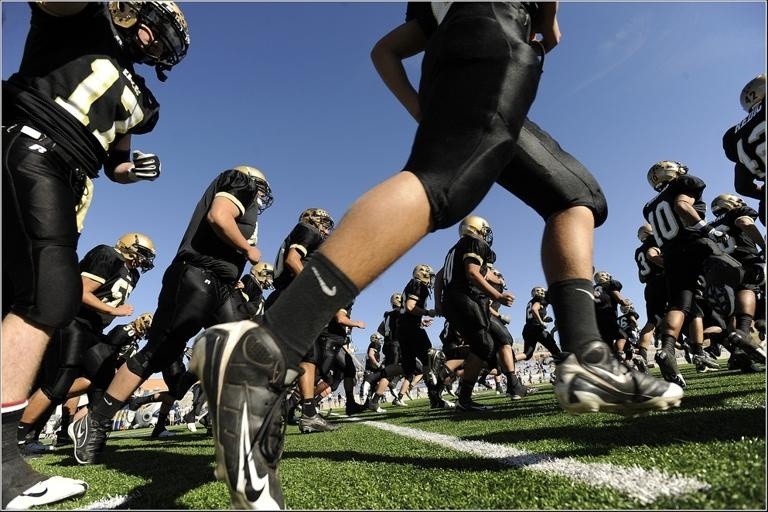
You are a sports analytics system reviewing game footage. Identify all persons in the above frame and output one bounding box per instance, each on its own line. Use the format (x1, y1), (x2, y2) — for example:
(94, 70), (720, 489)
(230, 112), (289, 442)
(357, 215), (566, 414)
(1, 1), (191, 510)
(185, 3), (687, 511)
(589, 73), (767, 392)
(14, 166), (364, 466)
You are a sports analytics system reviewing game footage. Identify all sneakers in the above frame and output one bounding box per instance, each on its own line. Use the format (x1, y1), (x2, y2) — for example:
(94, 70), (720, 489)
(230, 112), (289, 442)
(22, 442), (56, 454)
(188, 319), (305, 510)
(68, 412), (114, 466)
(2, 459), (88, 509)
(728, 329), (766, 362)
(552, 351), (685, 414)
(654, 349), (686, 388)
(614, 331), (766, 374)
(52, 431), (74, 447)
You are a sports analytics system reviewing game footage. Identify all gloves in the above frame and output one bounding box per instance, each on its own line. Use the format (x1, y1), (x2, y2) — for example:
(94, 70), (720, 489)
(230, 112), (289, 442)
(127, 149), (161, 183)
(693, 220), (730, 244)
